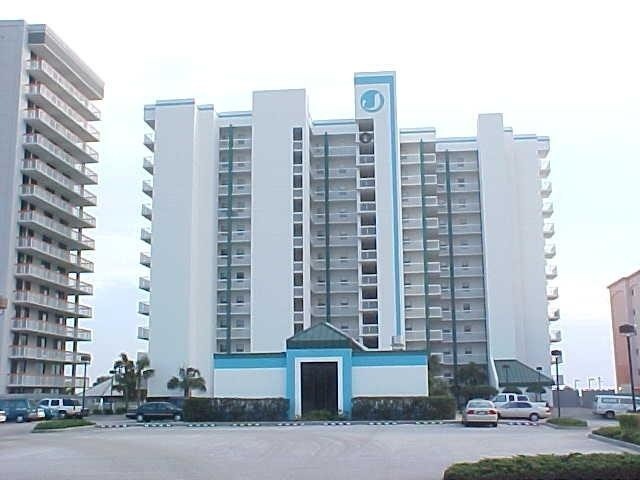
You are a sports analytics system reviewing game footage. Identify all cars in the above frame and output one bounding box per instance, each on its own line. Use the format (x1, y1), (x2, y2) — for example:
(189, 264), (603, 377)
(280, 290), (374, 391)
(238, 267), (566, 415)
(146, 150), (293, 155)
(0, 398), (89, 422)
(461, 392), (551, 428)
(126, 402), (183, 423)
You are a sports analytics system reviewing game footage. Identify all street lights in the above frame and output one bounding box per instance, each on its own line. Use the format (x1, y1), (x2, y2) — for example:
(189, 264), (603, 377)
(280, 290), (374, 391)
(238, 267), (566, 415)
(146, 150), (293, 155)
(81, 354), (91, 417)
(618, 323), (638, 413)
(551, 349), (562, 417)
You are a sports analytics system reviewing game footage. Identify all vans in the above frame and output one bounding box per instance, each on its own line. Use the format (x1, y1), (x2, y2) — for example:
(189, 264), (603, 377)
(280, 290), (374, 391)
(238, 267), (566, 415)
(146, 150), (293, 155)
(592, 394), (640, 420)
